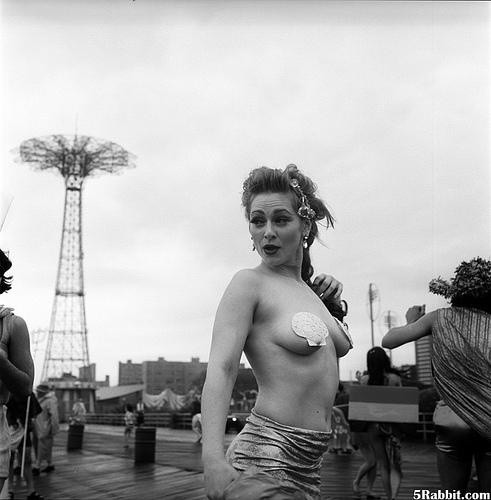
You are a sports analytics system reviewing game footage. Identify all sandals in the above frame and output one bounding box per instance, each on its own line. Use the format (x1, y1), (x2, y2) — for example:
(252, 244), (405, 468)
(6, 491), (14, 500)
(27, 491), (44, 500)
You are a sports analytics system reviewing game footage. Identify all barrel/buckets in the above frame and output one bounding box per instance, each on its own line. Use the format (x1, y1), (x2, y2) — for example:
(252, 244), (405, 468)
(67, 424), (84, 452)
(134, 427), (156, 465)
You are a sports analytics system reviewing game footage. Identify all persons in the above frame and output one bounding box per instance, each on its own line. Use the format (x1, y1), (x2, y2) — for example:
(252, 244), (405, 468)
(332, 346), (406, 499)
(198, 162), (358, 500)
(73, 398), (87, 423)
(123, 403), (137, 450)
(33, 383), (60, 476)
(383, 257), (491, 500)
(1, 248), (35, 500)
(6, 390), (45, 500)
(189, 392), (204, 444)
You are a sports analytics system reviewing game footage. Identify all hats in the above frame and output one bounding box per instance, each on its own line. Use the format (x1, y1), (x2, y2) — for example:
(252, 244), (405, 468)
(35, 384), (49, 393)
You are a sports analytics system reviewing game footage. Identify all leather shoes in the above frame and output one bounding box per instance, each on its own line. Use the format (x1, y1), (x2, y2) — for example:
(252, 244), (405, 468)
(42, 465), (55, 473)
(33, 468), (41, 476)
